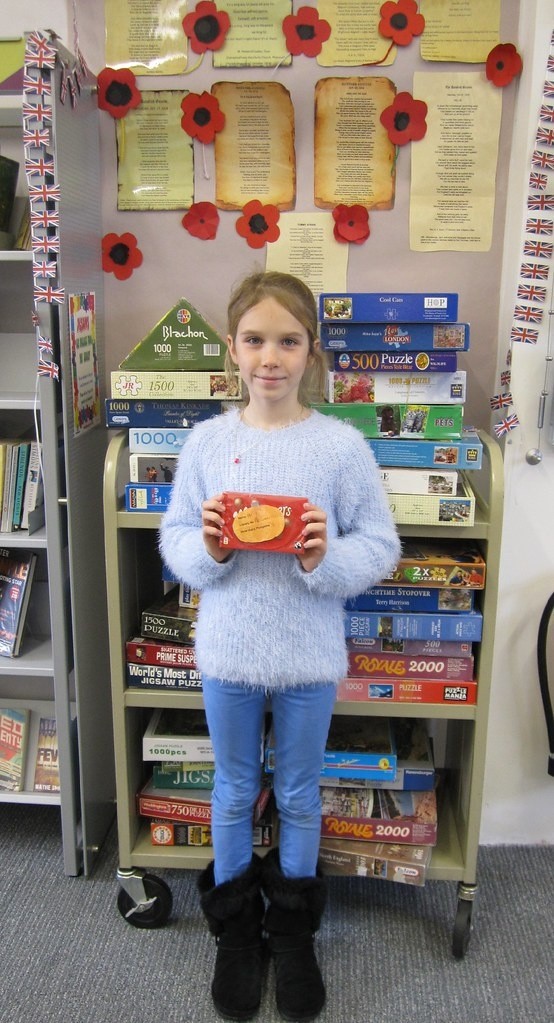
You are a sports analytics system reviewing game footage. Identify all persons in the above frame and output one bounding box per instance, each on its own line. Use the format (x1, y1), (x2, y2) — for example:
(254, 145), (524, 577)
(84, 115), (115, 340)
(157, 272), (404, 1023)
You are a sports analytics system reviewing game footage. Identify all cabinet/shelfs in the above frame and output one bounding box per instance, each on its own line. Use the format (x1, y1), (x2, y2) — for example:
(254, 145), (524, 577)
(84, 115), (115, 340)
(1, 31), (107, 889)
(99, 299), (511, 949)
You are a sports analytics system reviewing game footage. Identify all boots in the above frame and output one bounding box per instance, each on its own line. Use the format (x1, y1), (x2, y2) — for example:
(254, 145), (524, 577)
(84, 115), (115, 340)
(197, 850), (268, 1020)
(260, 847), (329, 1019)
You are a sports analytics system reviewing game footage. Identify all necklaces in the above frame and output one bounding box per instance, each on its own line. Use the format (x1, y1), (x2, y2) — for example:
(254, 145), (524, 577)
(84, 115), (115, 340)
(233, 400), (306, 465)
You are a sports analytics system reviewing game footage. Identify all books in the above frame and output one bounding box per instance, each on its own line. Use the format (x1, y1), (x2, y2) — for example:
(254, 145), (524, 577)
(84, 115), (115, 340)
(31, 716), (62, 792)
(0, 705), (31, 792)
(0, 547), (37, 658)
(0, 418), (43, 538)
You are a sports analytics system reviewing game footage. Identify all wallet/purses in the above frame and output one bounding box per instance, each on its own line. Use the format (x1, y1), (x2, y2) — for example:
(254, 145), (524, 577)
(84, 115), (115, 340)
(219, 491), (309, 555)
